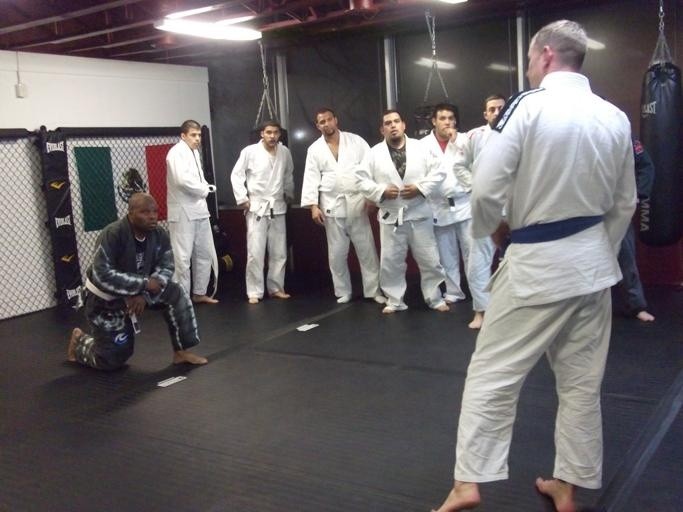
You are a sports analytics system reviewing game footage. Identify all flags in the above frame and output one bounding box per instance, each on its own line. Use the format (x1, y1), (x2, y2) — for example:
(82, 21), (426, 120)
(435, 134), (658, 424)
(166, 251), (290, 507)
(73, 143), (177, 231)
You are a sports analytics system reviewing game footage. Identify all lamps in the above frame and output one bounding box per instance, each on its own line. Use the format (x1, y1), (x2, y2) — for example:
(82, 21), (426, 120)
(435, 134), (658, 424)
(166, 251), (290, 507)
(153, 0), (263, 41)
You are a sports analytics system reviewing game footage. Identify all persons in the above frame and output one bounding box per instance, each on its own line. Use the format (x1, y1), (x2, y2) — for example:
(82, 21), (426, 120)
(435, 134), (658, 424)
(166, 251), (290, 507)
(67, 191), (208, 373)
(163, 119), (220, 306)
(228, 119), (295, 306)
(352, 109), (451, 315)
(618, 138), (657, 322)
(299, 107), (388, 305)
(419, 103), (473, 303)
(428, 19), (641, 512)
(453, 95), (509, 330)
